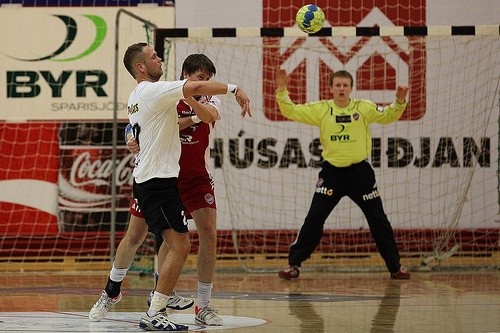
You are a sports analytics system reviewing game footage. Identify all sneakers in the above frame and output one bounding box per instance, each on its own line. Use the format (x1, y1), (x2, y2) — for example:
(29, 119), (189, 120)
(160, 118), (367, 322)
(89, 290), (122, 322)
(195, 306), (223, 326)
(139, 312), (188, 330)
(146, 293), (170, 317)
(147, 290), (193, 310)
(390, 268), (410, 279)
(280, 267), (300, 279)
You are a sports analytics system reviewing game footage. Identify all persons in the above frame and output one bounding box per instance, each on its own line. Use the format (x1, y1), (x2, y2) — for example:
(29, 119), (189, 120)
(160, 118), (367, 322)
(123, 42), (252, 331)
(88, 53), (224, 325)
(274, 68), (410, 280)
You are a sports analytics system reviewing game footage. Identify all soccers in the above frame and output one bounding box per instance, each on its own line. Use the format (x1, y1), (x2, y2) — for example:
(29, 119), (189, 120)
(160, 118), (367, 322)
(296, 4), (325, 34)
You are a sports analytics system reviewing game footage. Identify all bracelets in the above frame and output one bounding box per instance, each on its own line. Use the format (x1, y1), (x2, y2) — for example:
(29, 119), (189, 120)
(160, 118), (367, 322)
(192, 116), (200, 122)
(227, 83), (237, 96)
